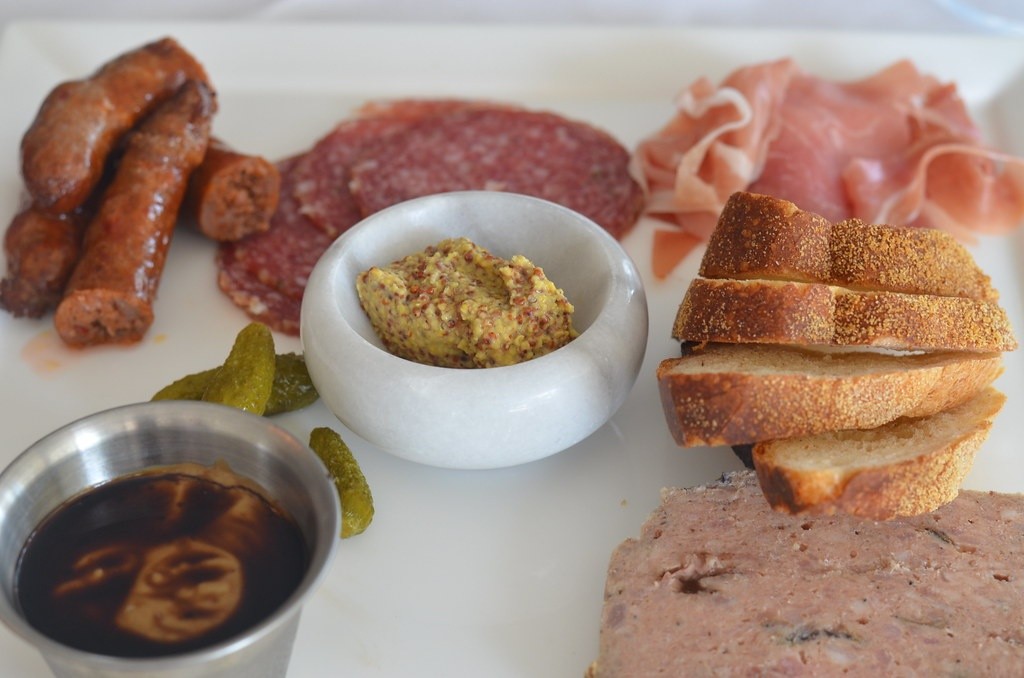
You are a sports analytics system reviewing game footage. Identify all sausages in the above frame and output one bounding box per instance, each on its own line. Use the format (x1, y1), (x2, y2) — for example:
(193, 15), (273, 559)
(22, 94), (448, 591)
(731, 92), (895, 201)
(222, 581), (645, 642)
(0, 36), (281, 347)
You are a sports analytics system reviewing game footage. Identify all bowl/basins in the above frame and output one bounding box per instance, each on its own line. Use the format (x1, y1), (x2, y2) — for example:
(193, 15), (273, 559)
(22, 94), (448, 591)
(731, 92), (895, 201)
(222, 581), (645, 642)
(0, 399), (341, 677)
(301, 191), (647, 470)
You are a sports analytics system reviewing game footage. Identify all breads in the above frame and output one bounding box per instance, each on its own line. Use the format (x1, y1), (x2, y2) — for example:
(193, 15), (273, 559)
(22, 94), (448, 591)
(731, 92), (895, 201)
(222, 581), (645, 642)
(655, 190), (1016, 523)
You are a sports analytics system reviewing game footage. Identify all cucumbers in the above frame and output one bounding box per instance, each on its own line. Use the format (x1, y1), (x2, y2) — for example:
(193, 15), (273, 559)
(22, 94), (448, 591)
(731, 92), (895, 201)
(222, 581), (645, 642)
(139, 322), (375, 538)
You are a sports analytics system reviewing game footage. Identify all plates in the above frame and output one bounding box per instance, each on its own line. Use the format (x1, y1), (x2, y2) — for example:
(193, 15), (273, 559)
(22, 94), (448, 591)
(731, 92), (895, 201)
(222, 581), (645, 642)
(0, 20), (1024, 678)
